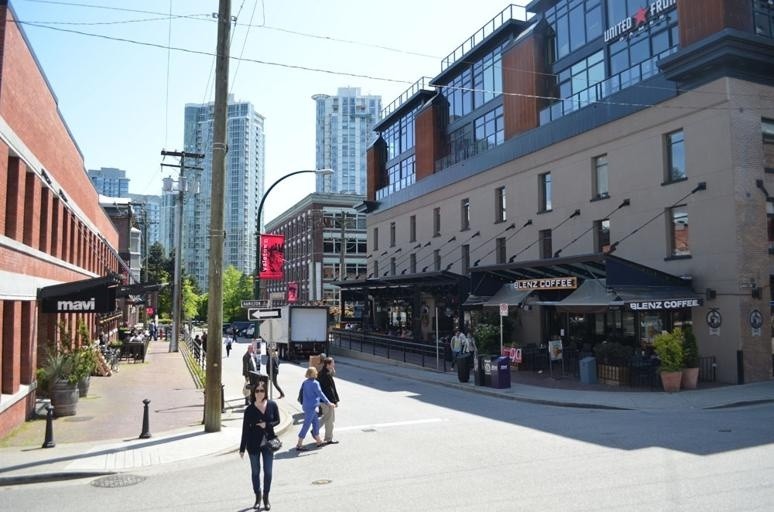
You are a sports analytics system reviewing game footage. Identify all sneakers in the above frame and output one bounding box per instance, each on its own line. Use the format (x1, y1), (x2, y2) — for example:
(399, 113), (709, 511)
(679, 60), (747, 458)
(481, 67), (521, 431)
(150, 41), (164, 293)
(278, 393), (285, 399)
(311, 430), (339, 446)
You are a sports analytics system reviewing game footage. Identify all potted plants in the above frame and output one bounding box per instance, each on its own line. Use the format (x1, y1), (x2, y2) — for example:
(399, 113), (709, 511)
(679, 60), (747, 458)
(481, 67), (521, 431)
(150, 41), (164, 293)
(652, 327), (682, 392)
(33, 320), (80, 415)
(65, 318), (96, 397)
(595, 343), (633, 386)
(679, 325), (700, 389)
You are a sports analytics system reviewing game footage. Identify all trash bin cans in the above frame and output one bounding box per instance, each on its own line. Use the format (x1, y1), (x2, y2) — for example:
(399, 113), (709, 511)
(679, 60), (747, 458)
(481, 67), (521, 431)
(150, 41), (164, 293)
(456, 352), (472, 382)
(475, 353), (511, 389)
(579, 356), (597, 385)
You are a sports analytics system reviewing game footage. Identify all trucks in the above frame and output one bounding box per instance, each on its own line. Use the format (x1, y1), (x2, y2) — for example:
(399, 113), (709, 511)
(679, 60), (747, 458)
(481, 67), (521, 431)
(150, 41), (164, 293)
(389, 311), (412, 330)
(258, 305), (328, 361)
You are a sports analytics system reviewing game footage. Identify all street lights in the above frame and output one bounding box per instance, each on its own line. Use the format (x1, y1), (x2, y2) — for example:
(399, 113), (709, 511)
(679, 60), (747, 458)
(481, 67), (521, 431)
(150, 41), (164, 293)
(253, 168), (335, 305)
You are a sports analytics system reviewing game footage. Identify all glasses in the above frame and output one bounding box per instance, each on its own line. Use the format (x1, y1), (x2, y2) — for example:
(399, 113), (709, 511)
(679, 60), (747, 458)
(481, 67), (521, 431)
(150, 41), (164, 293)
(255, 389), (264, 393)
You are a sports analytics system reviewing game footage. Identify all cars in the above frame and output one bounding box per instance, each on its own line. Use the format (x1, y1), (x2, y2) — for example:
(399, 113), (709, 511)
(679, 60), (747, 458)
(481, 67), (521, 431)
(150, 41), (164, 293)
(223, 320), (255, 338)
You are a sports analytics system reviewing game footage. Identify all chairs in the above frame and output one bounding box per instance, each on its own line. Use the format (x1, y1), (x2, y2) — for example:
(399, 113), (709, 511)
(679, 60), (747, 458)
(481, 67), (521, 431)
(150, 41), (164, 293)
(122, 329), (149, 363)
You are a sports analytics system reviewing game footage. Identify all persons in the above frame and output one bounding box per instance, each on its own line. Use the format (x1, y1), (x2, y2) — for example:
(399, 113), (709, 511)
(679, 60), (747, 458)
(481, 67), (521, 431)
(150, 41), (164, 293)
(251, 335), (258, 354)
(295, 365), (335, 451)
(242, 343), (259, 405)
(231, 325), (237, 343)
(223, 334), (232, 358)
(147, 320), (173, 341)
(265, 345), (285, 399)
(201, 331), (207, 358)
(448, 329), (467, 371)
(118, 327), (147, 361)
(256, 335), (262, 358)
(192, 334), (200, 363)
(464, 332), (477, 371)
(310, 357), (339, 444)
(313, 353), (336, 416)
(239, 381), (281, 511)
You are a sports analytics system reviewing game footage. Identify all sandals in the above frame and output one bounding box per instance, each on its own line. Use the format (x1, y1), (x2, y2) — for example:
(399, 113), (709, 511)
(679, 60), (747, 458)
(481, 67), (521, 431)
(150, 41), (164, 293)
(296, 444), (311, 451)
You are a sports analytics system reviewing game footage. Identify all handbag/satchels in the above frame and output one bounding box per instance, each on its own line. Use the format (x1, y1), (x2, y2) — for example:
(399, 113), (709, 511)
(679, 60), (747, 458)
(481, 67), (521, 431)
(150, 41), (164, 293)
(266, 437), (282, 453)
(241, 376), (252, 397)
(297, 382), (304, 405)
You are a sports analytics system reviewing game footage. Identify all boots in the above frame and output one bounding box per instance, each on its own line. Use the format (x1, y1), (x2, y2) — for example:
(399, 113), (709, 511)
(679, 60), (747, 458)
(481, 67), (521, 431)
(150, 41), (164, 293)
(262, 492), (272, 511)
(253, 488), (262, 509)
